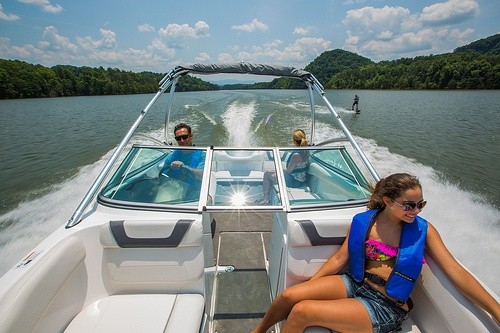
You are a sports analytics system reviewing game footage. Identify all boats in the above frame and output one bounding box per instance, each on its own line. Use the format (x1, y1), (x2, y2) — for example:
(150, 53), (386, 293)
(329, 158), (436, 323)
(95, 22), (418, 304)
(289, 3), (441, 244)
(1, 62), (500, 333)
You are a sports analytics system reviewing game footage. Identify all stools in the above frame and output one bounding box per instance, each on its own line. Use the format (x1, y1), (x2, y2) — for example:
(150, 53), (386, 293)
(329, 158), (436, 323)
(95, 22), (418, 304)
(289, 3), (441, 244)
(272, 191), (321, 205)
(268, 182), (313, 205)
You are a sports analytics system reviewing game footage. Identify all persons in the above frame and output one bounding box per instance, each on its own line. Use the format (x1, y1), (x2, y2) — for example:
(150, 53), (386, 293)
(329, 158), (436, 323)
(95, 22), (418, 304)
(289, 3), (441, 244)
(158, 123), (212, 205)
(250, 172), (500, 333)
(352, 95), (360, 111)
(255, 129), (309, 204)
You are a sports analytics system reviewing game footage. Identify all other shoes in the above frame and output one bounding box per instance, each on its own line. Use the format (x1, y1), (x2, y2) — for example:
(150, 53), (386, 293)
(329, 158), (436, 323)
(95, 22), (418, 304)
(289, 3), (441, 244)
(254, 200), (270, 205)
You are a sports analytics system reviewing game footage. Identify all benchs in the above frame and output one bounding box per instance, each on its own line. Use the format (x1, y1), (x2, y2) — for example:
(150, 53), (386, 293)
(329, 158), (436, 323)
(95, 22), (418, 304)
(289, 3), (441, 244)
(286, 218), (500, 333)
(0, 216), (208, 333)
(158, 150), (290, 183)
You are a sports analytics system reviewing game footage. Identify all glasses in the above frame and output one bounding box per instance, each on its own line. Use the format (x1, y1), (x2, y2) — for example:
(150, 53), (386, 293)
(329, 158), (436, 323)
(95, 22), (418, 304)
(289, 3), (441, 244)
(174, 134), (190, 140)
(391, 198), (427, 211)
